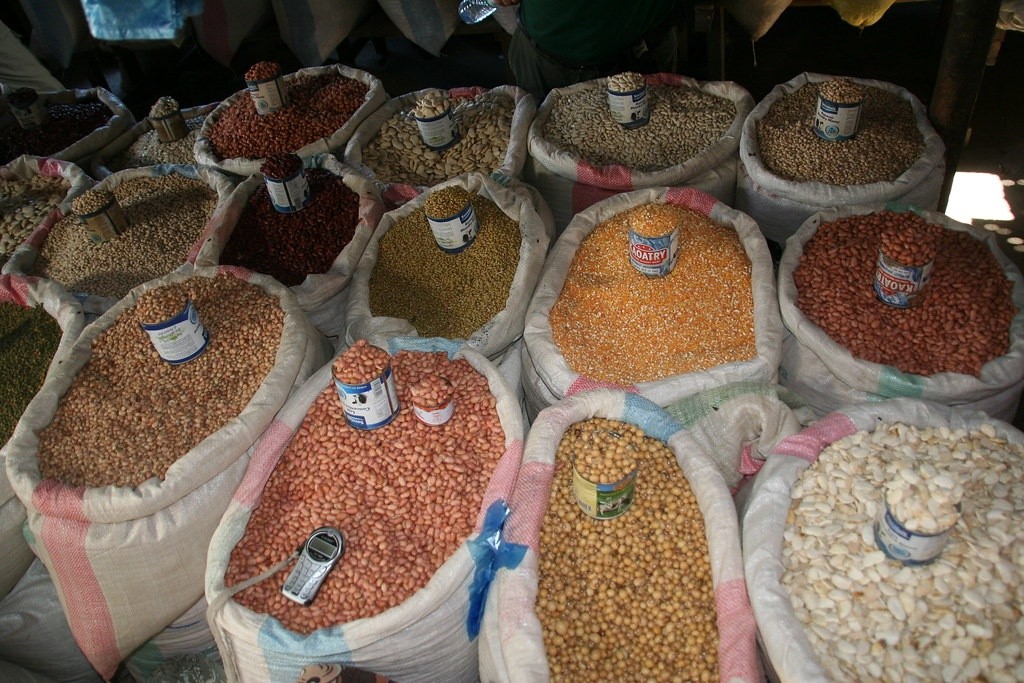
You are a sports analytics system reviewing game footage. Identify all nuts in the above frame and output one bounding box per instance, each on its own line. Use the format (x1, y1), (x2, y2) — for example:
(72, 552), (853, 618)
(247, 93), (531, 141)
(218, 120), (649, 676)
(224, 337), (506, 636)
(782, 424), (1024, 683)
(113, 59), (514, 189)
(793, 208), (1018, 378)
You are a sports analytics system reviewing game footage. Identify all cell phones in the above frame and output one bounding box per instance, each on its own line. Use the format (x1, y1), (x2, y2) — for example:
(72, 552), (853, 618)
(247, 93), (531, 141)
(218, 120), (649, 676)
(281, 526), (345, 607)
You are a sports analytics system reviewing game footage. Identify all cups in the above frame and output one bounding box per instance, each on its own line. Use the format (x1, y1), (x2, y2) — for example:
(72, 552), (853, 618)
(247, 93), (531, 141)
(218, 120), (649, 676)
(7, 88), (47, 129)
(629, 225), (679, 276)
(415, 105), (459, 149)
(874, 251), (933, 307)
(413, 376), (454, 426)
(573, 430), (636, 520)
(76, 193), (129, 244)
(140, 295), (210, 365)
(147, 110), (189, 144)
(245, 68), (290, 116)
(607, 85), (649, 130)
(875, 485), (965, 566)
(425, 190), (479, 253)
(264, 155), (311, 212)
(331, 344), (399, 430)
(813, 94), (863, 141)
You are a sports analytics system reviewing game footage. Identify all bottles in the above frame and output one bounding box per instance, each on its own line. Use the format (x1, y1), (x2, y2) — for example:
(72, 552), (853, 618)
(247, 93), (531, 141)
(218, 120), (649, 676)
(458, 0), (500, 24)
